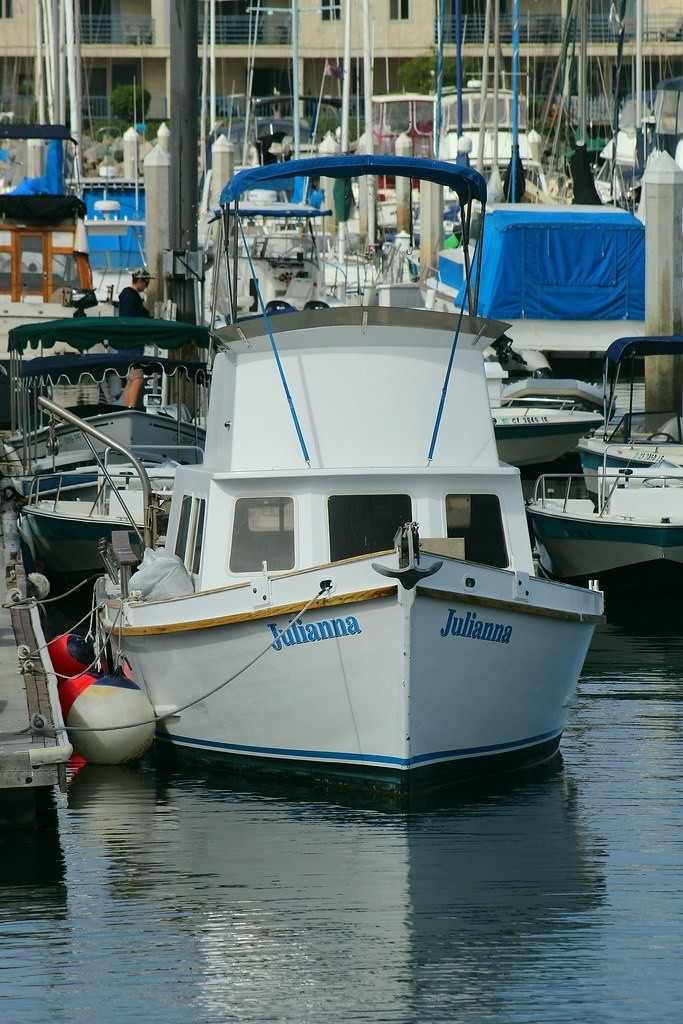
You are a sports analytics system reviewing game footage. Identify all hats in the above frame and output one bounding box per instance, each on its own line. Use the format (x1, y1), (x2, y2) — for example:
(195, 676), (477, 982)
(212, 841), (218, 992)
(132, 267), (156, 279)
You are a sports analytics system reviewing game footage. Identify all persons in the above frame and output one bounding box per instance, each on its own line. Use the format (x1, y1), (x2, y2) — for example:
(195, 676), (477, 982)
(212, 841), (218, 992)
(116, 268), (161, 318)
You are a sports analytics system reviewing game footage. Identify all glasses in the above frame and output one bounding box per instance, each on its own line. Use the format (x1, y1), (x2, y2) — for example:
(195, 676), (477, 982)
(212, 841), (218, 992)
(142, 280), (149, 285)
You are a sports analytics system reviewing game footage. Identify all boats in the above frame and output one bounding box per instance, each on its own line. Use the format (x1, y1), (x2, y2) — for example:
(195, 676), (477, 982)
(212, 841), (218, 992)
(1, 0), (683, 818)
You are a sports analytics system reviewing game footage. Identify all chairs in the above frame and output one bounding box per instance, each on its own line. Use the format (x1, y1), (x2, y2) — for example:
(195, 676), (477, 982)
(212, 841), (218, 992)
(659, 15), (683, 41)
(303, 300), (329, 311)
(121, 22), (143, 44)
(264, 300), (290, 312)
(536, 16), (554, 41)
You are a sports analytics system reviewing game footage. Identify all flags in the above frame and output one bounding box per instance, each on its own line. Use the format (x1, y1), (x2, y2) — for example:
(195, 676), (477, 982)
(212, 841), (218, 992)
(323, 56), (346, 81)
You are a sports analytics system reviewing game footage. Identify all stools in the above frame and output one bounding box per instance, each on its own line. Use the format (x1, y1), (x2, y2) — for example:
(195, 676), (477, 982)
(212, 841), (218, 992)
(274, 11), (290, 44)
